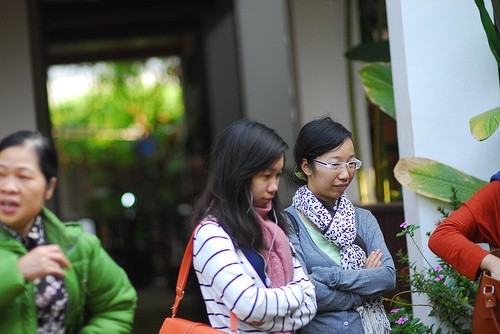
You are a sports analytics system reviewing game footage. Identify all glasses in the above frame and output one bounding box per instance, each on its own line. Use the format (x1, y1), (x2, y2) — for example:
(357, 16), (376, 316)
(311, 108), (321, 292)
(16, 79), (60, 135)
(312, 158), (362, 171)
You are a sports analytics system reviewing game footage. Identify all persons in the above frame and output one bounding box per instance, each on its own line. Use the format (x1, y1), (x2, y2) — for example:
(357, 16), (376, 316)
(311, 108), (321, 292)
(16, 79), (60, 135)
(0, 130), (138, 334)
(192, 121), (318, 334)
(282, 116), (396, 334)
(428, 170), (500, 334)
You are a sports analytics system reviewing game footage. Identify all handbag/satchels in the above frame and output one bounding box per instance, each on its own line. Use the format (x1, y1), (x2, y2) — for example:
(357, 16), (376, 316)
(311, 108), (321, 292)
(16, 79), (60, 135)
(159, 220), (238, 334)
(473, 249), (500, 334)
